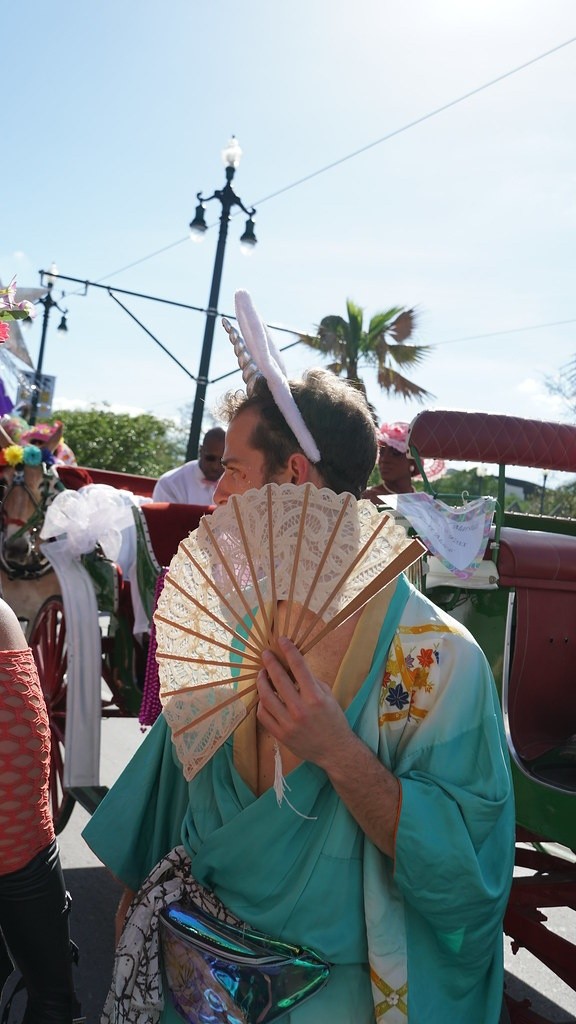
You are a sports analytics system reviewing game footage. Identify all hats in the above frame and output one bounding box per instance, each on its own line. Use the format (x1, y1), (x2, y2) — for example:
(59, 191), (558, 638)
(375, 422), (445, 479)
(20, 424), (64, 459)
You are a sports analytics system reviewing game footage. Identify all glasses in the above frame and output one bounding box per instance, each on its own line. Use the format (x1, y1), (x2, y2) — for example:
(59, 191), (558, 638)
(30, 439), (44, 445)
(201, 447), (223, 462)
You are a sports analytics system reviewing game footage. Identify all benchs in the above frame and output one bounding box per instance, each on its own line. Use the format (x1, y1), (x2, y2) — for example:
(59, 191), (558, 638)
(131, 502), (215, 629)
(55, 465), (158, 617)
(406, 407), (576, 592)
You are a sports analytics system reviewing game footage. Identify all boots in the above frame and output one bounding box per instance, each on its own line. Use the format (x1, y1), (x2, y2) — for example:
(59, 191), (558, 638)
(0, 837), (81, 1024)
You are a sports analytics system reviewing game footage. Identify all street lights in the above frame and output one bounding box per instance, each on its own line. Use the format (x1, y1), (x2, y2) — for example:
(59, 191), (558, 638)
(9, 263), (70, 424)
(184, 134), (258, 462)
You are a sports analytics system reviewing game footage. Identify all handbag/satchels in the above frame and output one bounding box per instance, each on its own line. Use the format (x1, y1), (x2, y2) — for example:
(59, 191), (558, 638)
(158, 891), (331, 1024)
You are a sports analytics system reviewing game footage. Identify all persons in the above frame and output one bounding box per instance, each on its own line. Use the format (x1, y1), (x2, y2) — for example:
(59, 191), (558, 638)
(0, 592), (77, 1024)
(362, 418), (446, 504)
(79, 369), (515, 1024)
(153, 426), (227, 504)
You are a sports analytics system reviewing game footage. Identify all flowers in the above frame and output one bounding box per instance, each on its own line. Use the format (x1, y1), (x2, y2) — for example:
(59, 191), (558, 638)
(2, 444), (23, 467)
(0, 450), (9, 466)
(40, 446), (56, 470)
(23, 445), (43, 467)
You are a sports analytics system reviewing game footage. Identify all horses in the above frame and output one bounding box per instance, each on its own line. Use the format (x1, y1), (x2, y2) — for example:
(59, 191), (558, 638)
(0, 424), (61, 641)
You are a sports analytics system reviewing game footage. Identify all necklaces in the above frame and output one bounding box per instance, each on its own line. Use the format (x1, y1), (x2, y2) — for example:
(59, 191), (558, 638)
(382, 481), (417, 495)
